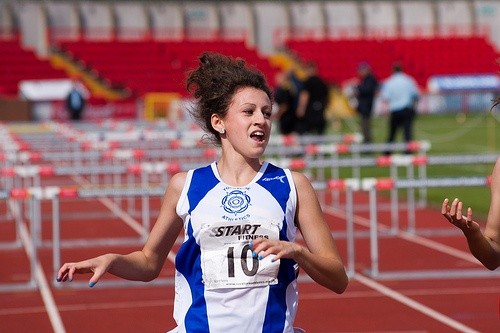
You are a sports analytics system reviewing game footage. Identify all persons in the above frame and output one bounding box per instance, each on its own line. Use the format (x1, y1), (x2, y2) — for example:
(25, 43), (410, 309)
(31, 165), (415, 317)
(348, 62), (380, 156)
(56, 51), (349, 333)
(442, 96), (500, 272)
(381, 65), (422, 156)
(271, 59), (331, 159)
(64, 85), (85, 122)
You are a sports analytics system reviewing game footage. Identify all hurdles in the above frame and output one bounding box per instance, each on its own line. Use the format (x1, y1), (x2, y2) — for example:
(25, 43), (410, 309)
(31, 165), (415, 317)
(0, 118), (431, 292)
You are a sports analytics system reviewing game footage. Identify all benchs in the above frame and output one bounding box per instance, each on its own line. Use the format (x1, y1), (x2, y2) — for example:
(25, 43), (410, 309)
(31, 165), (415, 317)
(0, 26), (500, 102)
